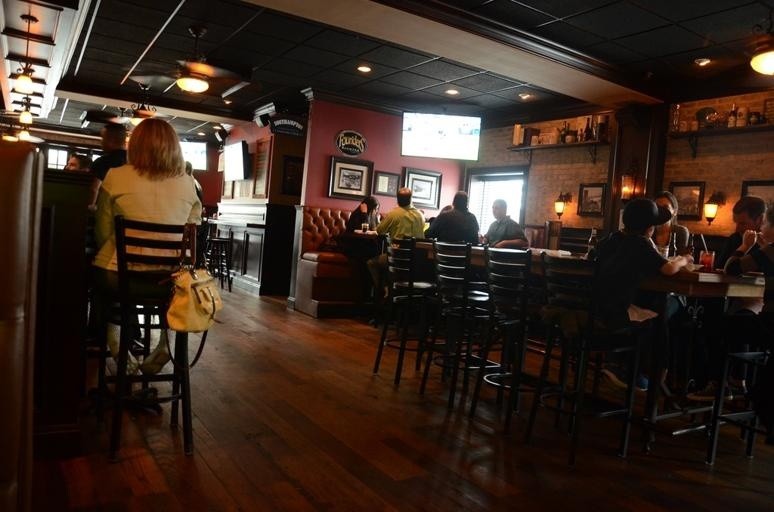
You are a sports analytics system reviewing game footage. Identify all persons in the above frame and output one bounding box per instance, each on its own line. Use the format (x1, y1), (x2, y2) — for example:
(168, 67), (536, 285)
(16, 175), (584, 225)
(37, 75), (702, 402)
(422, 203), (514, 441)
(685, 200), (773, 405)
(86, 121), (128, 214)
(573, 196), (695, 392)
(672, 193), (769, 417)
(86, 118), (203, 376)
(338, 186), (531, 299)
(637, 190), (695, 261)
(63, 153), (93, 174)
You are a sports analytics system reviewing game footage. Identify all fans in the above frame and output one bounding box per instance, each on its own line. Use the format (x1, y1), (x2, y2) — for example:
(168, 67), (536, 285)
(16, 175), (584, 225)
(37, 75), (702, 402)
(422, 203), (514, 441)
(128, 23), (262, 103)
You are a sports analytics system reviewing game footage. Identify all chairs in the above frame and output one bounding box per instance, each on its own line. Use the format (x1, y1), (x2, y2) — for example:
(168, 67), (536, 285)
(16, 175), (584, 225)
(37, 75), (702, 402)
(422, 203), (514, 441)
(94, 212), (213, 465)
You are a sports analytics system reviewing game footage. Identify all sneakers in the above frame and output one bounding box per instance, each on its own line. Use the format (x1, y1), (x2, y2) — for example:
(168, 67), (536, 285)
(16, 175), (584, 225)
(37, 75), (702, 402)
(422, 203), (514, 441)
(687, 386), (733, 401)
(604, 364), (630, 388)
(112, 349), (138, 375)
(141, 345), (170, 374)
(633, 376), (649, 391)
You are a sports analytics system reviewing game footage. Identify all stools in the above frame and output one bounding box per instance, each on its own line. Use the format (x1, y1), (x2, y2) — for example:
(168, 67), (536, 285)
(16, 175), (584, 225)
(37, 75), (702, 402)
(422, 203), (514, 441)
(206, 233), (233, 293)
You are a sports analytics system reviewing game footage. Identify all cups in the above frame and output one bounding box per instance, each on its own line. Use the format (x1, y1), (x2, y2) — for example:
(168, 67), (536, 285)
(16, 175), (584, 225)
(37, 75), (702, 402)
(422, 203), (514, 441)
(698, 250), (715, 273)
(362, 223), (369, 234)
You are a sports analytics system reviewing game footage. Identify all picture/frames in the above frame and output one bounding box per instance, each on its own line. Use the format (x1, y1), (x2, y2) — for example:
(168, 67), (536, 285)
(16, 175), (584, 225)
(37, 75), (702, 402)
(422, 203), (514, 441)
(576, 182), (606, 217)
(326, 155), (443, 213)
(668, 178), (706, 222)
(740, 179), (774, 207)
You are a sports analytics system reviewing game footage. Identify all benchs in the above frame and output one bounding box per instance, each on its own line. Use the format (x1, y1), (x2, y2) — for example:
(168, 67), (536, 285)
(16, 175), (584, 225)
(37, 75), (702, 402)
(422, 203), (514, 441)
(292, 201), (390, 321)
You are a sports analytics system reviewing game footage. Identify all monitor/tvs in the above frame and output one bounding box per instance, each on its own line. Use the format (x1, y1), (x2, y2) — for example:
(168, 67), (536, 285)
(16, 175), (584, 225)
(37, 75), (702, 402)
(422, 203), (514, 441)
(400, 109), (482, 162)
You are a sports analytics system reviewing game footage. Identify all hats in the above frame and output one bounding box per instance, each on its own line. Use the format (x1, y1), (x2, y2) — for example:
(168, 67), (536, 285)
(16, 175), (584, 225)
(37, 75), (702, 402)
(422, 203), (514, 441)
(622, 198), (671, 227)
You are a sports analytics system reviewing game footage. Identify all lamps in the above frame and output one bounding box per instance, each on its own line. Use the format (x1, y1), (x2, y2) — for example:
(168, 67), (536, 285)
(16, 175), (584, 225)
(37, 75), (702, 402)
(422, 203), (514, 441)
(553, 191), (572, 221)
(173, 71), (211, 96)
(703, 190), (726, 226)
(743, 28), (774, 78)
(13, 58), (35, 126)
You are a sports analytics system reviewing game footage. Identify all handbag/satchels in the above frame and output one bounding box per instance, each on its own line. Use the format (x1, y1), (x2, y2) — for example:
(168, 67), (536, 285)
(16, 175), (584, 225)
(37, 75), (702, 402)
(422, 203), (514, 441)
(164, 267), (222, 368)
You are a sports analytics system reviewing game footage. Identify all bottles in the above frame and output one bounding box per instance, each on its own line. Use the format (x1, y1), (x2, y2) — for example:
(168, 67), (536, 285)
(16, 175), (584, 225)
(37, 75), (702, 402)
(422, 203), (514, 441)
(668, 231), (678, 259)
(727, 101), (765, 129)
(672, 103), (699, 132)
(559, 117), (592, 143)
(587, 227), (600, 252)
